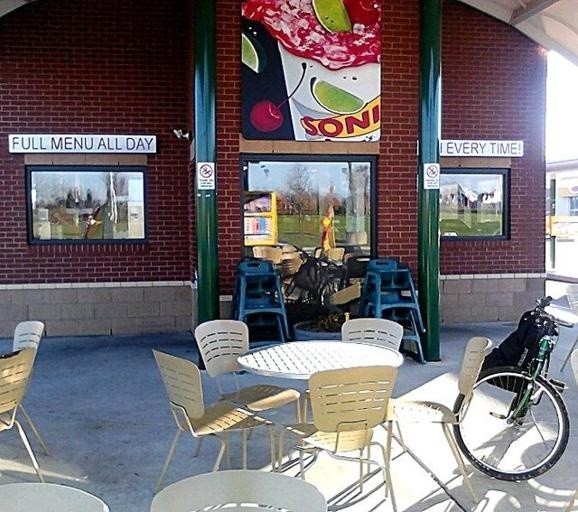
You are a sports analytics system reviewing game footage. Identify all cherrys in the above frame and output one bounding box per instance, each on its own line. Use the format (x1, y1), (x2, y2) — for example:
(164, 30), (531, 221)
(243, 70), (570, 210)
(249, 62), (309, 133)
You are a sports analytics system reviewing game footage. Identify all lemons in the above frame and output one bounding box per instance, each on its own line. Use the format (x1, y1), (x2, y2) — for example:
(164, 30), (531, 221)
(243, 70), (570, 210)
(311, 0), (353, 34)
(311, 76), (367, 114)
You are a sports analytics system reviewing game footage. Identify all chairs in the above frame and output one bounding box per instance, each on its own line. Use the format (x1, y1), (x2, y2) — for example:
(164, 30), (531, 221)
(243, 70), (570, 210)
(251, 239), (368, 313)
(7, 318), (48, 457)
(280, 365), (402, 512)
(0, 478), (115, 512)
(298, 317), (406, 477)
(147, 347), (278, 478)
(193, 319), (307, 477)
(0, 343), (46, 487)
(149, 467), (330, 511)
(379, 332), (493, 506)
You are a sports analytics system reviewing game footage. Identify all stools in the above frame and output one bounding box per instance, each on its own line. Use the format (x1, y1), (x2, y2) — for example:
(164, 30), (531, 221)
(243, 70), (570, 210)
(226, 257), (439, 368)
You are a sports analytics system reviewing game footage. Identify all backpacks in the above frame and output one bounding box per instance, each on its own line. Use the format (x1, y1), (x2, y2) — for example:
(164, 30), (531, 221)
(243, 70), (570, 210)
(480, 311), (551, 392)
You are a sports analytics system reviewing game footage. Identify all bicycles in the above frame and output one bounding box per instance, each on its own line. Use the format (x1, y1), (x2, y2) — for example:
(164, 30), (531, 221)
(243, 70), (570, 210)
(451, 296), (573, 482)
(279, 244), (349, 309)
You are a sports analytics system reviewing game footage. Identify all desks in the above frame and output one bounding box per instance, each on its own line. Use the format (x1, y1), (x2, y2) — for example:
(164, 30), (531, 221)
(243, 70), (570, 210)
(234, 336), (405, 482)
